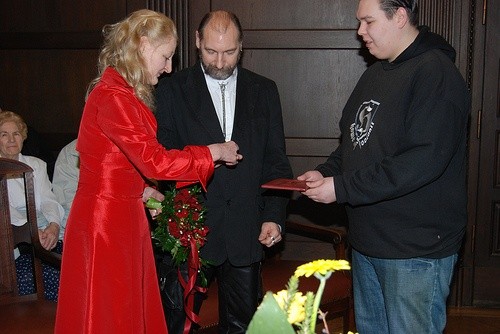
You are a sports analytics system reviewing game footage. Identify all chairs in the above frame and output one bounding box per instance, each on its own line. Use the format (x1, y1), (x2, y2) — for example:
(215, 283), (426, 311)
(0, 157), (64, 334)
(261, 222), (353, 334)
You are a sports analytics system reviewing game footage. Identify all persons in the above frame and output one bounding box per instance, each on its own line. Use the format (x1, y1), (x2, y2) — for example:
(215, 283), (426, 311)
(297, 0), (470, 334)
(153, 10), (294, 334)
(52, 138), (81, 241)
(0, 111), (64, 302)
(54, 8), (242, 334)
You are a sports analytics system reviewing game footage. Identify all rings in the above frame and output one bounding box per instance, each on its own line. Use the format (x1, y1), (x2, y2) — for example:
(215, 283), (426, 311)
(270, 236), (275, 244)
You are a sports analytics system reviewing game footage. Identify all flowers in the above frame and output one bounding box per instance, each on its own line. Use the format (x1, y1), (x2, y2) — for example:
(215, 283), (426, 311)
(246, 260), (360, 334)
(145, 188), (214, 289)
